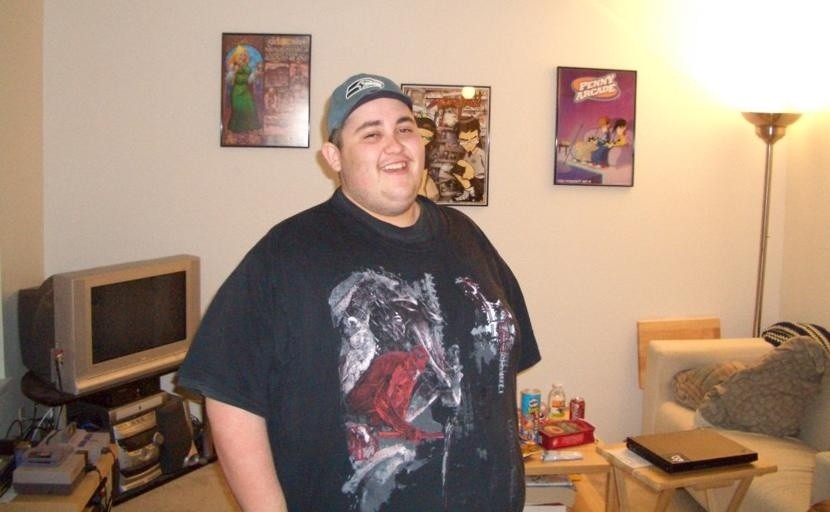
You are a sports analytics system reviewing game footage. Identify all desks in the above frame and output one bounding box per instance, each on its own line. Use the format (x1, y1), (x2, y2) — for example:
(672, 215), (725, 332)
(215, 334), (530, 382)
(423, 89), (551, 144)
(516, 433), (611, 512)
(0, 444), (119, 512)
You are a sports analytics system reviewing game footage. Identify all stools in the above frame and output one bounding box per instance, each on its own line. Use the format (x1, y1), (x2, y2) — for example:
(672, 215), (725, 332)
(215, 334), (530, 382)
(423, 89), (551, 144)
(596, 442), (777, 512)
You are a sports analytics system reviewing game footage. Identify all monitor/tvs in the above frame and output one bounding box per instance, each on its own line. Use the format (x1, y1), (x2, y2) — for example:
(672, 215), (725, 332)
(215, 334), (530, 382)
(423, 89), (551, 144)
(17, 256), (200, 395)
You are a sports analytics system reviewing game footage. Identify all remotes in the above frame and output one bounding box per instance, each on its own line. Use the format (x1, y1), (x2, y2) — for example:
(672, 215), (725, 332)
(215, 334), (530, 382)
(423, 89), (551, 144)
(542, 451), (583, 461)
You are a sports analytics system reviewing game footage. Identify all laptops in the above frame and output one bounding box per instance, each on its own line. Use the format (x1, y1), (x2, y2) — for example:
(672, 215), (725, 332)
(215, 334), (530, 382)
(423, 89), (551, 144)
(626, 426), (759, 475)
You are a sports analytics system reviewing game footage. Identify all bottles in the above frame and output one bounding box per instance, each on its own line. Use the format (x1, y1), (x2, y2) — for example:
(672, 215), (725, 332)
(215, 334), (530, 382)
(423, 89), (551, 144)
(547, 382), (567, 419)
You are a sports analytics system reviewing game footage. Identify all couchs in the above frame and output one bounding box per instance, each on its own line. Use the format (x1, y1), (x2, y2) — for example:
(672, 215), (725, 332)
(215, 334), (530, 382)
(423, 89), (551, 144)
(642, 334), (830, 512)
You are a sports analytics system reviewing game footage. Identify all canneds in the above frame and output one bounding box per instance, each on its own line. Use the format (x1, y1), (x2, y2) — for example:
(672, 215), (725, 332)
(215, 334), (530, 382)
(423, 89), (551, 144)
(570, 397), (585, 420)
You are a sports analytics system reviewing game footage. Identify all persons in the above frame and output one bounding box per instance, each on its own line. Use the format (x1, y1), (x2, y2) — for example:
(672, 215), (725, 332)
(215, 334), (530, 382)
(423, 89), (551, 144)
(176, 72), (542, 512)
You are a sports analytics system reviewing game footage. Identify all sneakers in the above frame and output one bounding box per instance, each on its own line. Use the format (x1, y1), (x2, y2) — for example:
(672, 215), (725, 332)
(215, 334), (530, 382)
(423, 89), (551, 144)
(454, 186), (476, 201)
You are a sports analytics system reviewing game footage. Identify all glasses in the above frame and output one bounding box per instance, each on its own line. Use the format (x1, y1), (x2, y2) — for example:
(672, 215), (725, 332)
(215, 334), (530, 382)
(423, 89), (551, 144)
(458, 138), (480, 145)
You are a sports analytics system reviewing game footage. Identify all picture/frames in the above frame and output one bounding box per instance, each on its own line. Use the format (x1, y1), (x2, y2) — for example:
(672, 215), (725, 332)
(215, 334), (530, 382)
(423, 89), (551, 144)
(554, 64), (637, 188)
(398, 84), (492, 208)
(220, 33), (311, 149)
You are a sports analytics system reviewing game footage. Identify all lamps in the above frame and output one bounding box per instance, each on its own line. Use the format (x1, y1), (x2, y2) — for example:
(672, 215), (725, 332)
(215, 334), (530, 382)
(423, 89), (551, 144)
(689, 2), (830, 338)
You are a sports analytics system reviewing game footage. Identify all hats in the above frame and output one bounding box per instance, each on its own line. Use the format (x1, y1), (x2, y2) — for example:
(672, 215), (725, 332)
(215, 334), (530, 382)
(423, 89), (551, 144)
(327, 73), (412, 141)
(417, 118), (434, 132)
